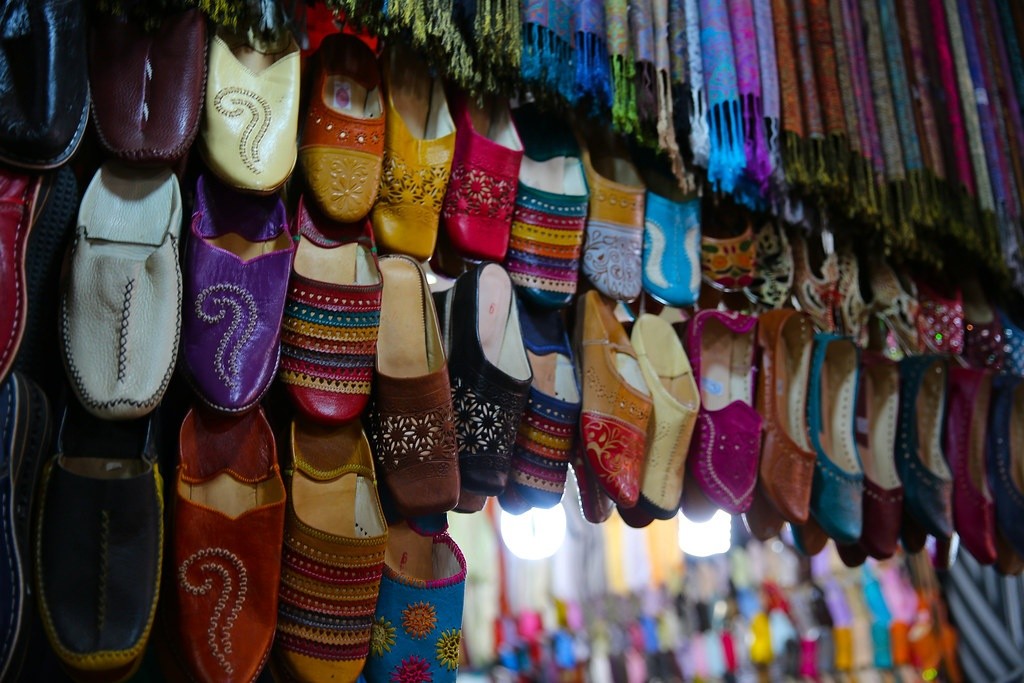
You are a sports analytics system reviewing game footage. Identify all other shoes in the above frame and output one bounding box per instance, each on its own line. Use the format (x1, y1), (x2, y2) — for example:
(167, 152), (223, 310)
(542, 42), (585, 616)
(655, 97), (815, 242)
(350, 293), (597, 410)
(0, 0), (1024, 683)
